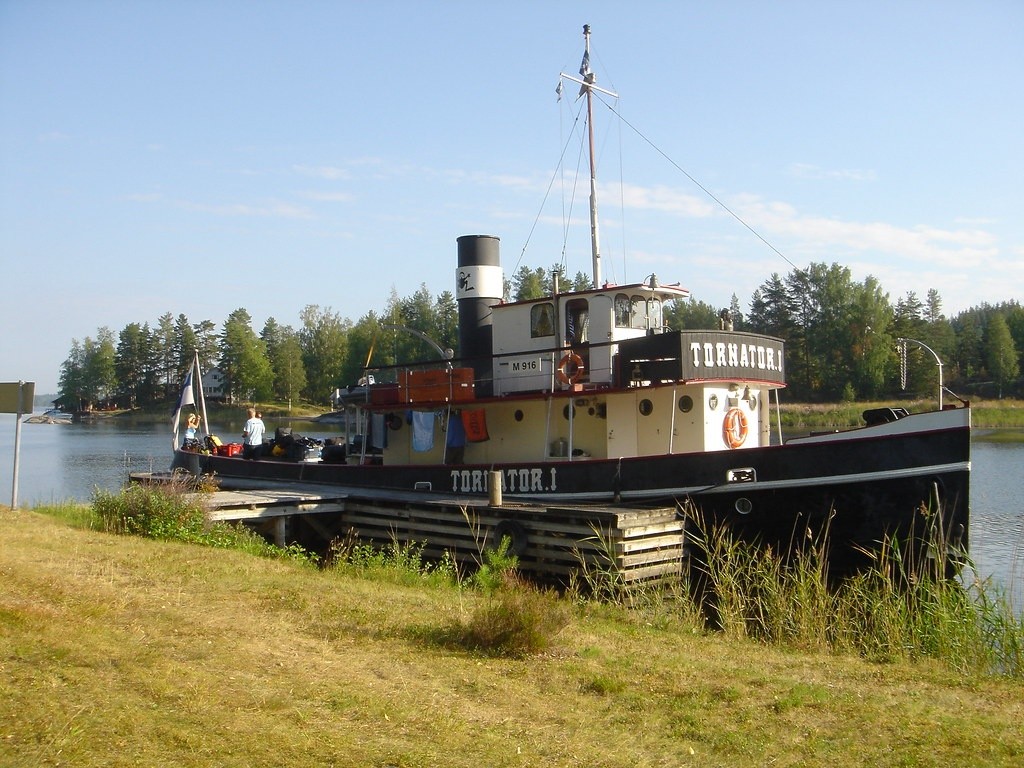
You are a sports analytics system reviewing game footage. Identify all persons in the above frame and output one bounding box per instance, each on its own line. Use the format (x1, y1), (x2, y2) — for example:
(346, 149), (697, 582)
(242, 408), (265, 459)
(719, 308), (734, 332)
(255, 412), (261, 420)
(183, 413), (201, 447)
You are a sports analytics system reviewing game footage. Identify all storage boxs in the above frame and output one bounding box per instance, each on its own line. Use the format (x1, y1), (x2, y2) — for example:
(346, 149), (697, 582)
(220, 443), (240, 455)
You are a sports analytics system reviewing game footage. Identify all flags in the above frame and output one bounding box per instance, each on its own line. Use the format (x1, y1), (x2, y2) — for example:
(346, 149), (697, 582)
(170, 357), (196, 451)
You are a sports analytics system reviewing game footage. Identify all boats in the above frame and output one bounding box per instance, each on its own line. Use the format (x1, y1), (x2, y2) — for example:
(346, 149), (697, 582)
(170, 23), (971, 593)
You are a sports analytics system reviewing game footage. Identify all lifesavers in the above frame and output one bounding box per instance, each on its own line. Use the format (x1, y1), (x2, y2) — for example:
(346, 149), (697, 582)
(557, 352), (585, 385)
(723, 409), (749, 448)
